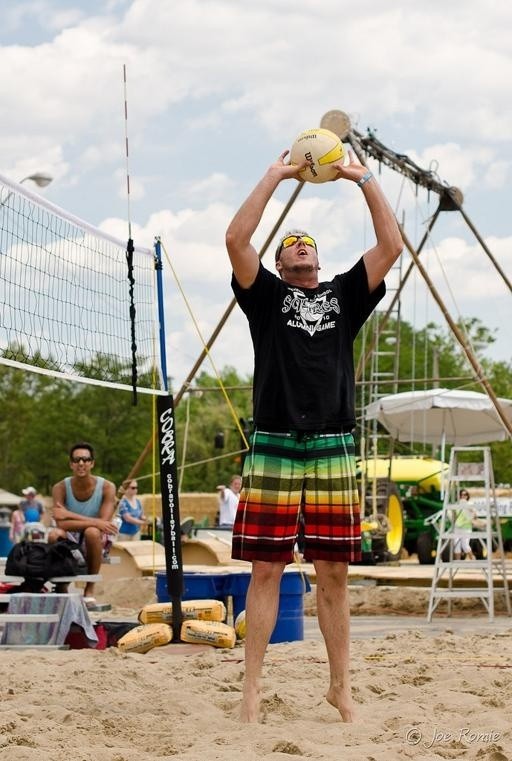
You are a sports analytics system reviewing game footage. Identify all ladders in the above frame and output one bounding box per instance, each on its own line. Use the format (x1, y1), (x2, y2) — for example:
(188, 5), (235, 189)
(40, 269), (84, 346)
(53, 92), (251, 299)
(426, 447), (511, 621)
(365, 209), (405, 484)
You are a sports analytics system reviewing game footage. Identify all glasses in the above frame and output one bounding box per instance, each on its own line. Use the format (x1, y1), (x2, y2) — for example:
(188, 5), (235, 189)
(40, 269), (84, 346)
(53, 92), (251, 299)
(71, 456), (92, 463)
(128, 486), (139, 489)
(278, 234), (317, 261)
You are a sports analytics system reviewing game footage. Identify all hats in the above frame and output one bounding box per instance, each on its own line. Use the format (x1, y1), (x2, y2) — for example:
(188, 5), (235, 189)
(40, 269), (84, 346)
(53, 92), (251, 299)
(21, 486), (36, 496)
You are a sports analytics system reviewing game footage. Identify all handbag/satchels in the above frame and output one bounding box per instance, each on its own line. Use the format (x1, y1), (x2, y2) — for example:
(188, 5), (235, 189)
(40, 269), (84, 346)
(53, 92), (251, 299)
(4, 535), (88, 577)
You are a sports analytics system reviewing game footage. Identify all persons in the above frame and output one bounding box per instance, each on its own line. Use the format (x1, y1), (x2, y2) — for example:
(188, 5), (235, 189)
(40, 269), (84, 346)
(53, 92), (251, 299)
(452, 490), (478, 560)
(16, 485), (45, 522)
(225, 148), (405, 727)
(116, 478), (149, 541)
(50, 442), (120, 608)
(8, 508), (24, 544)
(215, 474), (241, 526)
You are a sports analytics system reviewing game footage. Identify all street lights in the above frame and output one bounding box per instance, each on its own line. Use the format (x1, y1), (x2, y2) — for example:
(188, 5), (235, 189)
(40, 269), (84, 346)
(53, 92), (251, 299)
(387, 330), (441, 391)
(0, 171), (53, 206)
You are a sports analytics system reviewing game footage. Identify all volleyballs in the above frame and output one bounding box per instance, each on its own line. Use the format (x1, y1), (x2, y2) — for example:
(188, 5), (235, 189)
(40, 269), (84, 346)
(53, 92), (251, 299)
(290, 129), (345, 184)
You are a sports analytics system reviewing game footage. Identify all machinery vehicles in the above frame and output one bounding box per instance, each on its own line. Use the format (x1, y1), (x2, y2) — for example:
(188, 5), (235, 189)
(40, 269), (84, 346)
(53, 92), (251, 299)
(353, 452), (512, 570)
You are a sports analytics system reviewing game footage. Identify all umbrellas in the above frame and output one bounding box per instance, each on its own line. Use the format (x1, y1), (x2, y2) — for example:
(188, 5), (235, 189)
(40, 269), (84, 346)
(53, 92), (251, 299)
(368, 388), (509, 502)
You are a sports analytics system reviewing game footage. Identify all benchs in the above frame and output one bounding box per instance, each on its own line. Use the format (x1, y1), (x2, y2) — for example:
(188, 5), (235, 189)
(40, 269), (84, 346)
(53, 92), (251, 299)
(0, 555), (122, 651)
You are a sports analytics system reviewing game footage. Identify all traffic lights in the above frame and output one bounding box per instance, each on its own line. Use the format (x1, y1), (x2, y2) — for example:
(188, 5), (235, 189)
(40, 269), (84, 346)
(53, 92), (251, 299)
(215, 432), (224, 449)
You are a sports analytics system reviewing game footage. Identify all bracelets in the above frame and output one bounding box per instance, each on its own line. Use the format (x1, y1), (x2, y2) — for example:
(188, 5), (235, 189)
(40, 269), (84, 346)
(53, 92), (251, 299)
(357, 171), (373, 187)
